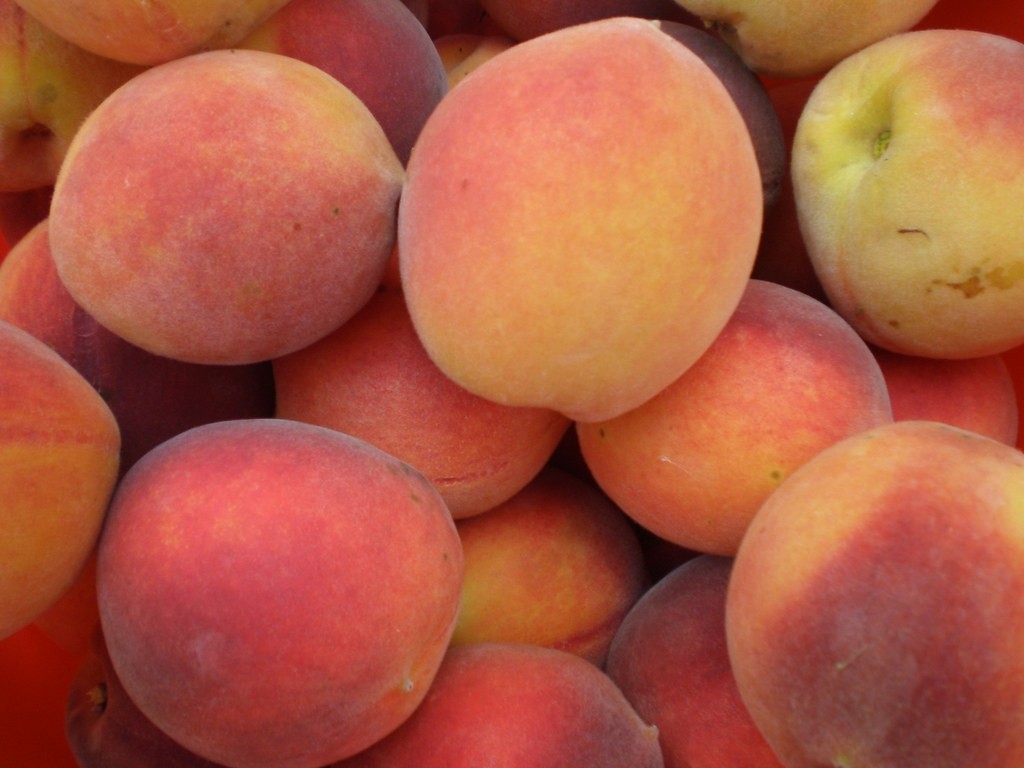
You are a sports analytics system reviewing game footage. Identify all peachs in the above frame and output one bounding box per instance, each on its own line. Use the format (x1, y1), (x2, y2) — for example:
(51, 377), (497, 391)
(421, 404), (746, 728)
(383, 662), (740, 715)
(0, 0), (1024, 768)
